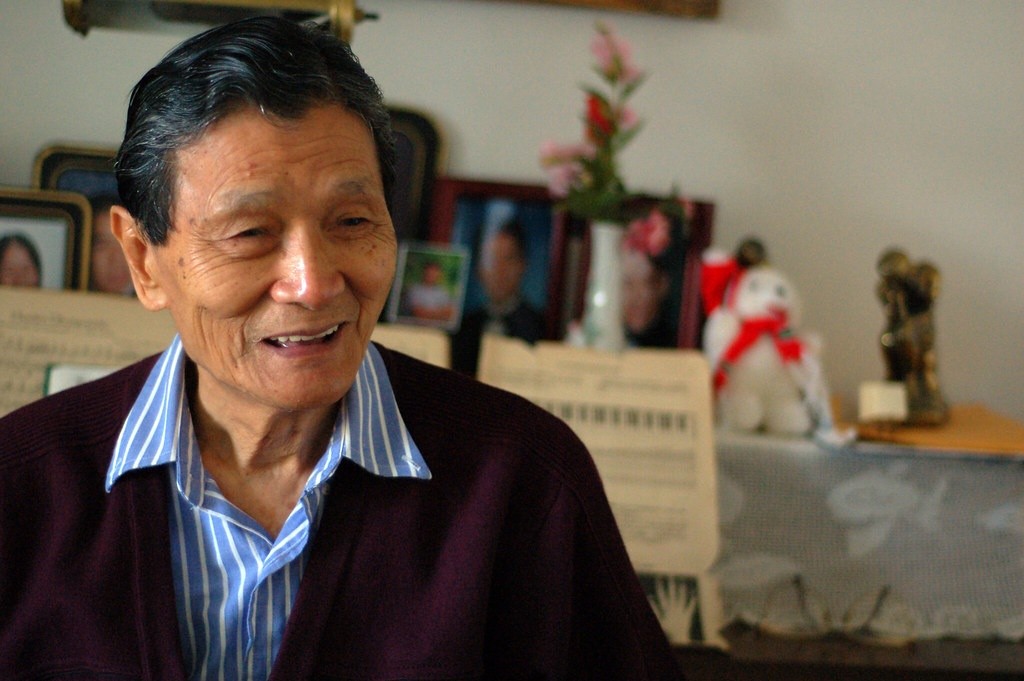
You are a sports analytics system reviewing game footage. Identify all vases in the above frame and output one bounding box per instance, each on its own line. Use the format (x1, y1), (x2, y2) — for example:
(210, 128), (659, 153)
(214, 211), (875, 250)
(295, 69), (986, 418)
(580, 222), (631, 349)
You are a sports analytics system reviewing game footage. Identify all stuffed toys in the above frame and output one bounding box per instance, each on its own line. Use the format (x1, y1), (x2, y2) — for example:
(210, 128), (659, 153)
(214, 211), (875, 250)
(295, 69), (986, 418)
(694, 236), (855, 451)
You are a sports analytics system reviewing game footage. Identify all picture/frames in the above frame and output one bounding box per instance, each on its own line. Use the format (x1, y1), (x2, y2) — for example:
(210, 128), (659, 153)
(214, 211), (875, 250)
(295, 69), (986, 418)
(1, 143), (711, 350)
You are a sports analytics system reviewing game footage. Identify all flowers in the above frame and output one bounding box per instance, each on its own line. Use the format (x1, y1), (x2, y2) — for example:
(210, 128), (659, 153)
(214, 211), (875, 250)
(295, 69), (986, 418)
(534, 21), (691, 257)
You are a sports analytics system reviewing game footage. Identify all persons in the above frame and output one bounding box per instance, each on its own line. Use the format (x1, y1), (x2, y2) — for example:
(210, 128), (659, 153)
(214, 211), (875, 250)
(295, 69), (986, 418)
(404, 252), (454, 321)
(76, 189), (133, 297)
(1, 15), (688, 681)
(613, 202), (677, 341)
(1, 233), (45, 290)
(460, 210), (551, 339)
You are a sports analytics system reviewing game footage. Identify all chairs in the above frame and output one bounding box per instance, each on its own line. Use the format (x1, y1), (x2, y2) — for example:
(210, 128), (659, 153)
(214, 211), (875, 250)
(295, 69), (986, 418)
(473, 333), (727, 650)
